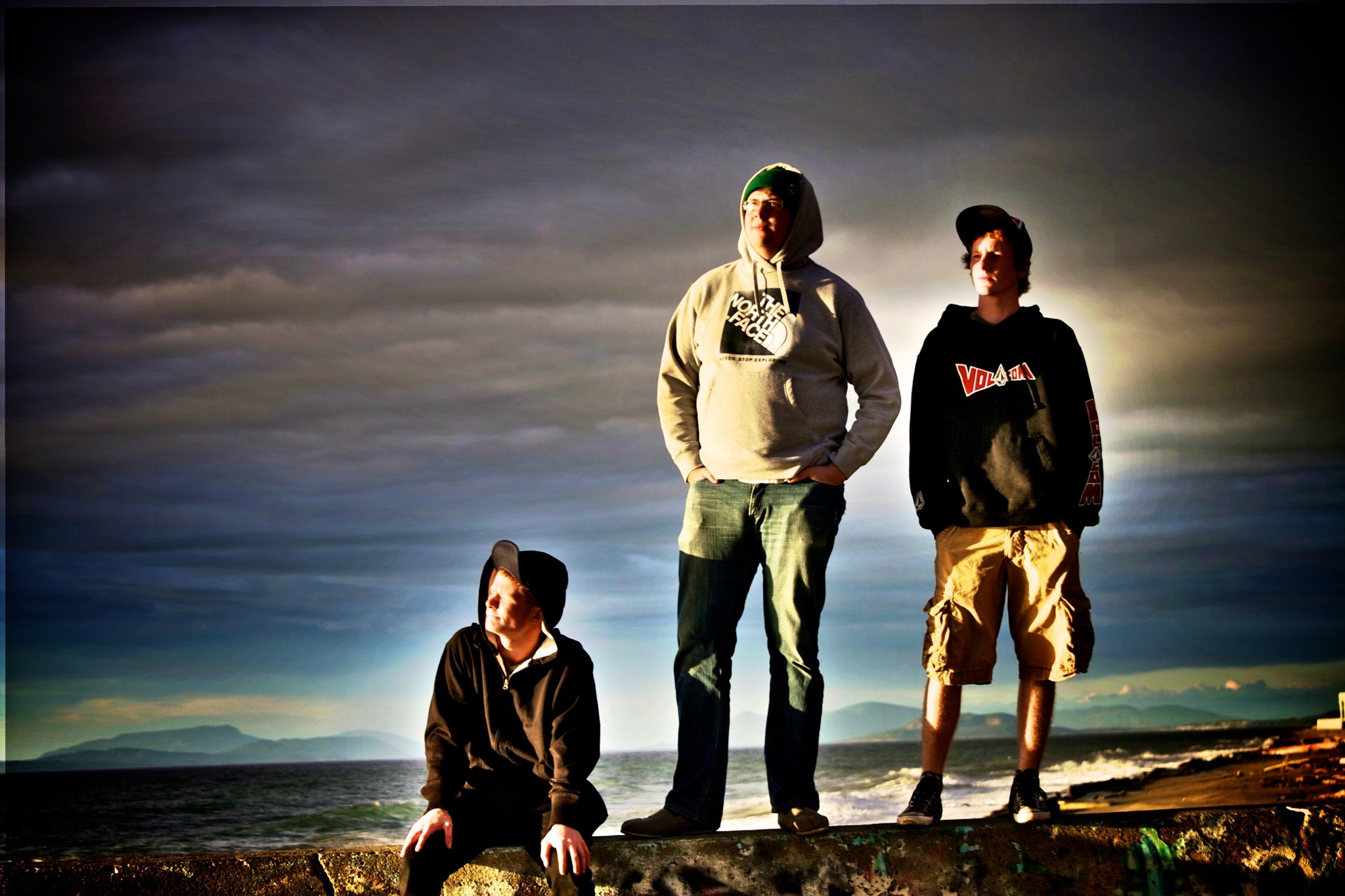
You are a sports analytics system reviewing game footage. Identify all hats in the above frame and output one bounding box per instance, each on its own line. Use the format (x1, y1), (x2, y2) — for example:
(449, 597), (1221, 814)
(492, 539), (569, 622)
(741, 166), (800, 213)
(955, 204), (1032, 279)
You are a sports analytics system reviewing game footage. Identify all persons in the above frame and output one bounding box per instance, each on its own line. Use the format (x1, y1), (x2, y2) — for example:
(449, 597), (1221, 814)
(897, 203), (1103, 827)
(620, 158), (900, 839)
(400, 540), (608, 896)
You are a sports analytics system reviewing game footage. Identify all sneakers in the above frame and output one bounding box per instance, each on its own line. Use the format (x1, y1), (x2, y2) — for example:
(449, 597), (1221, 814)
(896, 771), (943, 823)
(777, 796), (830, 834)
(620, 806), (721, 835)
(1009, 768), (1051, 823)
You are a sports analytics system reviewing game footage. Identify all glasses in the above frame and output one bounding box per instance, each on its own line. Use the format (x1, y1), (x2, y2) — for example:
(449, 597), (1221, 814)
(741, 199), (784, 210)
